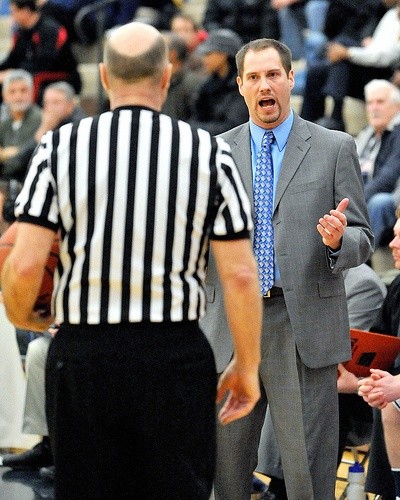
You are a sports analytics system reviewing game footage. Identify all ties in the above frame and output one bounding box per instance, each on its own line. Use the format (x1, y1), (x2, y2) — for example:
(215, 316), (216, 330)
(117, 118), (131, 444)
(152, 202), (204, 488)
(253, 130), (276, 295)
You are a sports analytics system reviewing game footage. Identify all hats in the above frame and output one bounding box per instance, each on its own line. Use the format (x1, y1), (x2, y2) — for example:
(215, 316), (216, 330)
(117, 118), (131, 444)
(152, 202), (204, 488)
(198, 27), (241, 57)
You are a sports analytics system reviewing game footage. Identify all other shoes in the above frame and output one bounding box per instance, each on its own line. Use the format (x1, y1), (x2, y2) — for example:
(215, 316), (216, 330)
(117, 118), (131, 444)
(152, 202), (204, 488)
(259, 489), (287, 500)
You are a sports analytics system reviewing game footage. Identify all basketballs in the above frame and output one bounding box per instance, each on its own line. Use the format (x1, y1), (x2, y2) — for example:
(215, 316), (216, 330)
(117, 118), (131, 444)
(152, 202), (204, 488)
(0, 219), (59, 314)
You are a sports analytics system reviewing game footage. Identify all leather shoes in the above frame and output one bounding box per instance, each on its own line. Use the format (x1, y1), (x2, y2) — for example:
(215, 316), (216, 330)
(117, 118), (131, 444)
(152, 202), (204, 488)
(40, 465), (55, 481)
(0, 442), (50, 468)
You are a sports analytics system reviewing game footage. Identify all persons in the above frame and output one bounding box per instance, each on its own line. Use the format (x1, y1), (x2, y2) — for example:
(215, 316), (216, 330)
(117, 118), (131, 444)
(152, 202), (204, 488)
(0, 0), (400, 226)
(354, 79), (400, 252)
(2, 337), (52, 470)
(343, 261), (384, 334)
(194, 37), (372, 500)
(0, 19), (263, 500)
(255, 203), (399, 500)
(357, 369), (400, 500)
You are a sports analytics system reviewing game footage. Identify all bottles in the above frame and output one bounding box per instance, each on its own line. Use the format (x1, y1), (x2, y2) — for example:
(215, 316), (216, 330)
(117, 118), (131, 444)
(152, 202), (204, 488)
(346, 462), (366, 500)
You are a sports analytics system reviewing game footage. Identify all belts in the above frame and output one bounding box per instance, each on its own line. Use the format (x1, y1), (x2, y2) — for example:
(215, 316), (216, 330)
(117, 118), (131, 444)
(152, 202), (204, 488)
(263, 287), (284, 299)
